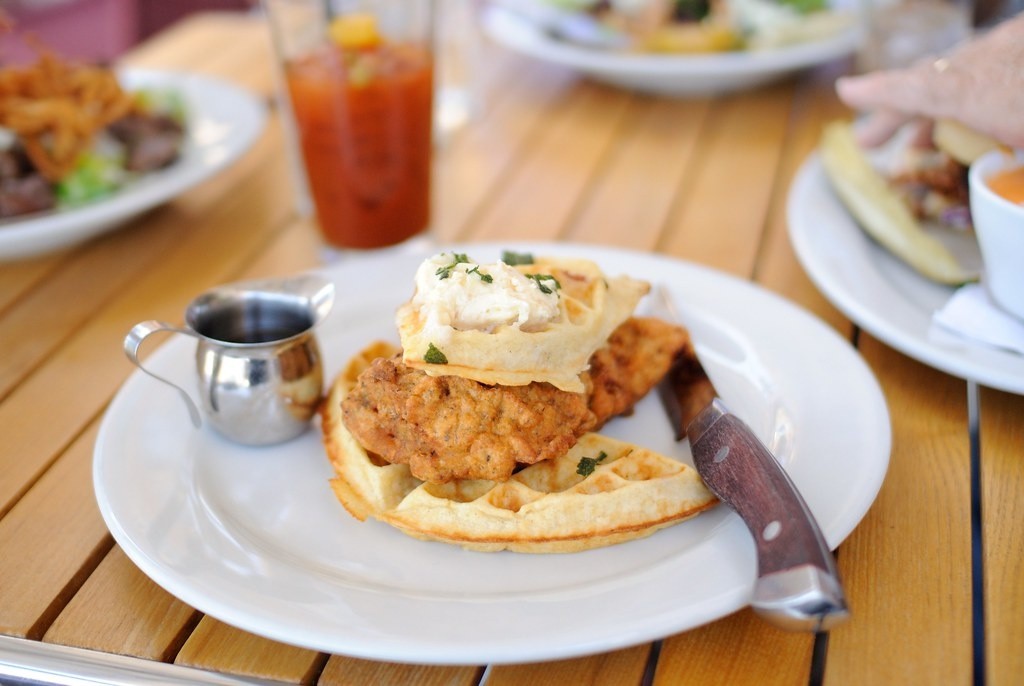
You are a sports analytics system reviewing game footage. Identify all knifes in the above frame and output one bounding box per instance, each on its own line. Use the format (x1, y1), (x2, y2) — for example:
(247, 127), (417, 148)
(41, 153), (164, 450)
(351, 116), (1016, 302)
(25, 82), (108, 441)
(658, 347), (848, 632)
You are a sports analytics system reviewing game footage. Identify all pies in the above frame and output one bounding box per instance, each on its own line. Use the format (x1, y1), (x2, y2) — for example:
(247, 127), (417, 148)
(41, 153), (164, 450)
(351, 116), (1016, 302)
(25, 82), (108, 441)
(318, 342), (714, 550)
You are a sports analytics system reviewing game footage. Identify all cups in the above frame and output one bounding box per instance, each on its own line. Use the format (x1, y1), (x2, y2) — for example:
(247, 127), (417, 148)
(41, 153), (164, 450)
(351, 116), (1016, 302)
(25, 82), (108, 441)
(271, 0), (442, 249)
(122, 274), (332, 444)
(968, 148), (1024, 322)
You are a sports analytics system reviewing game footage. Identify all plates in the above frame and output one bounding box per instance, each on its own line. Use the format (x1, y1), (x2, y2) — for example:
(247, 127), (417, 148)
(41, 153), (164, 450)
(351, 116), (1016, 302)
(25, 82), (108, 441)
(0, 66), (267, 259)
(786, 113), (1024, 396)
(477, 1), (873, 98)
(92, 242), (893, 661)
